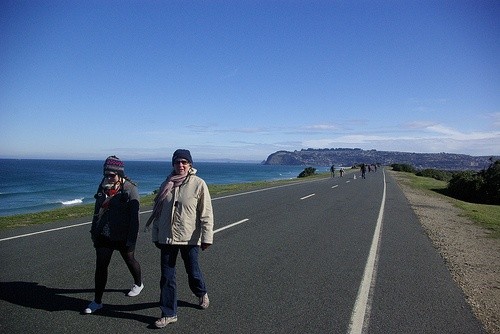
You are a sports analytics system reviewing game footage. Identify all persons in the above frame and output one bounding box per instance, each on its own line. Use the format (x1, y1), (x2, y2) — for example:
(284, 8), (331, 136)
(85, 155), (144, 313)
(330, 165), (335, 177)
(374, 164), (377, 173)
(340, 168), (344, 176)
(361, 163), (366, 179)
(368, 165), (371, 173)
(144, 148), (214, 327)
(351, 165), (357, 169)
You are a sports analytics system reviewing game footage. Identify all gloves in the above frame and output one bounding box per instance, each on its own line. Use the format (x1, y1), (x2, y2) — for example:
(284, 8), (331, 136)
(155, 241), (160, 248)
(200, 242), (210, 250)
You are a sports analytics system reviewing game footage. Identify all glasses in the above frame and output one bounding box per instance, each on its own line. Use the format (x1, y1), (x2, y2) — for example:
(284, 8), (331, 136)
(174, 160), (189, 165)
(103, 173), (118, 178)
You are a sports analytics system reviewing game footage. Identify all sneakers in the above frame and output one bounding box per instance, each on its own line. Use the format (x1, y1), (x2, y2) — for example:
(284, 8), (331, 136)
(128, 282), (144, 297)
(199, 295), (209, 310)
(85, 301), (103, 314)
(155, 315), (177, 328)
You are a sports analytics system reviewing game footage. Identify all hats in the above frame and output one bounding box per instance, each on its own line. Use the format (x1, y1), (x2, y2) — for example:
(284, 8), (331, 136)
(173, 149), (192, 163)
(104, 156), (124, 172)
